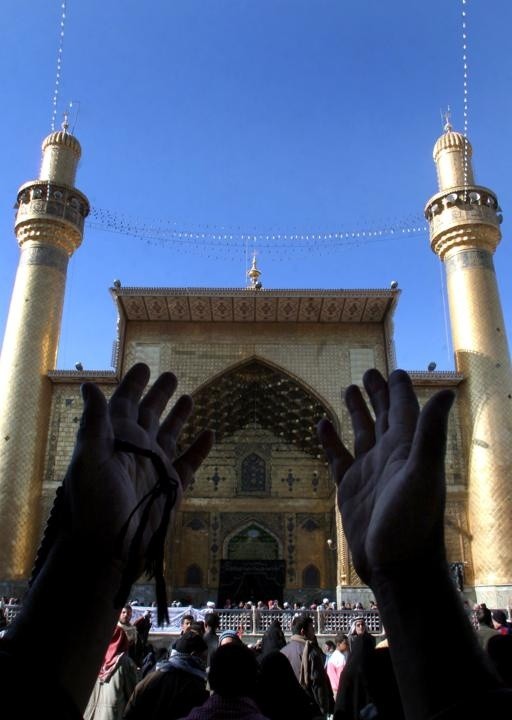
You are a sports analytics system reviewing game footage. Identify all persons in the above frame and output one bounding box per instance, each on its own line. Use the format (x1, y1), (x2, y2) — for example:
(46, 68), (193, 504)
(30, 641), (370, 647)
(2, 595), (25, 637)
(226, 599), (377, 610)
(81, 600), (269, 718)
(472, 603), (510, 652)
(0, 360), (510, 720)
(0, 363), (512, 720)
(254, 617), (406, 718)
